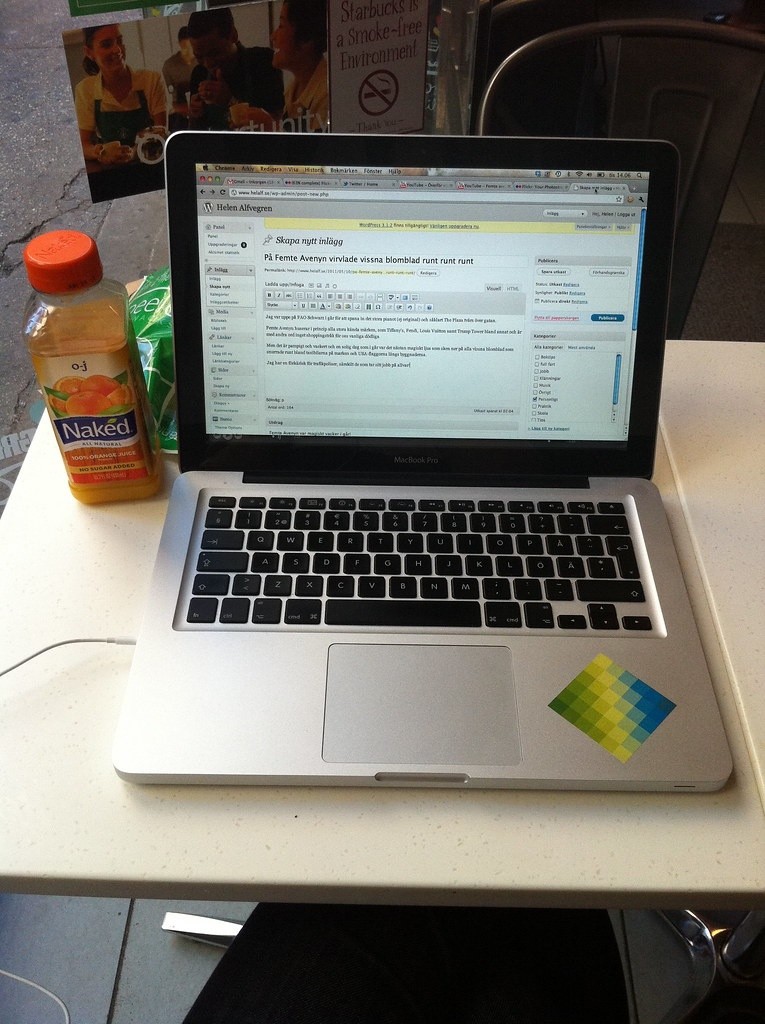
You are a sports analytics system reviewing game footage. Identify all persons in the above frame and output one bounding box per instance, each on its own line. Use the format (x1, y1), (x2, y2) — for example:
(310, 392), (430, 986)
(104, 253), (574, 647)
(162, 25), (199, 94)
(173, 8), (283, 130)
(74, 23), (167, 169)
(229, 0), (331, 136)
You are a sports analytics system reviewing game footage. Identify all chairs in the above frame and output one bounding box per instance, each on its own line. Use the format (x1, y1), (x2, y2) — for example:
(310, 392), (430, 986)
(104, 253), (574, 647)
(470, 0), (765, 340)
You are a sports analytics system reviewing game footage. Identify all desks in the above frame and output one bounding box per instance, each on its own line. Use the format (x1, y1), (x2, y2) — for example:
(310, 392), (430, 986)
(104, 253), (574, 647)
(0, 335), (765, 912)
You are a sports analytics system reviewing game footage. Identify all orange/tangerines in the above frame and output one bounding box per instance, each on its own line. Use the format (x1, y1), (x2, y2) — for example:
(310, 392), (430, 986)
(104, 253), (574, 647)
(49, 375), (134, 417)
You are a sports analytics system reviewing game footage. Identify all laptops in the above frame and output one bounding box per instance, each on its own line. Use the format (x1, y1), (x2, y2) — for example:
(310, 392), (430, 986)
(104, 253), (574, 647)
(115, 129), (732, 794)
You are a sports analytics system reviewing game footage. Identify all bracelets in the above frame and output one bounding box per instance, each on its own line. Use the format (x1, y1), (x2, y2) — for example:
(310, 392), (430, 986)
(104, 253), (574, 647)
(96, 145), (105, 161)
